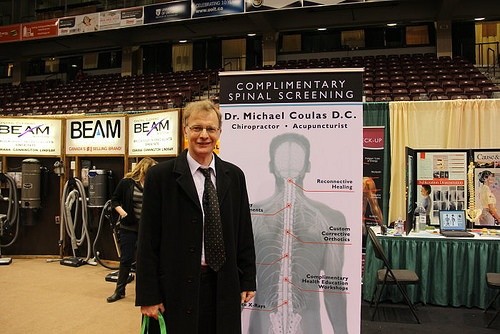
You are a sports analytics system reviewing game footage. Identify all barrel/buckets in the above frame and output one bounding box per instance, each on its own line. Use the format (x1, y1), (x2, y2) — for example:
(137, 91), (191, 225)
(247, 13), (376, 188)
(87, 168), (106, 208)
(22, 158), (41, 208)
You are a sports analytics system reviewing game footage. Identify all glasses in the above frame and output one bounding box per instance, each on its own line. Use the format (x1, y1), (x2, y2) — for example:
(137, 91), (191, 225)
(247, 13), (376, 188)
(187, 124), (220, 133)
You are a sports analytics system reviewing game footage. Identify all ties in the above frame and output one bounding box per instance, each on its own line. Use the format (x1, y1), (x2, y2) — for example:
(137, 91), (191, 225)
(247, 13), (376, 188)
(197, 167), (227, 272)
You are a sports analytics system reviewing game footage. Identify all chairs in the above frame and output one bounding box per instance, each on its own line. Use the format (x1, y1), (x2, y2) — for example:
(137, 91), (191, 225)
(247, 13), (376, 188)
(482, 273), (499, 328)
(367, 229), (421, 324)
(1, 54), (500, 116)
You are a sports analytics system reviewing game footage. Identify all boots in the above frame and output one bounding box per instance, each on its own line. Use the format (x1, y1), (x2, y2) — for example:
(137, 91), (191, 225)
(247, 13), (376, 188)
(107, 266), (130, 302)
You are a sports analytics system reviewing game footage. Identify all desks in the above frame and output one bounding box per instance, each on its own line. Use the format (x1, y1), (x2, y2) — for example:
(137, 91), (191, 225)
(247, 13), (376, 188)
(363, 226), (499, 310)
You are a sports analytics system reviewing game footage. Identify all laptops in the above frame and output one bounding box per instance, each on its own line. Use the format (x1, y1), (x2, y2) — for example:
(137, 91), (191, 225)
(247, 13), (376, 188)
(439, 210), (474, 237)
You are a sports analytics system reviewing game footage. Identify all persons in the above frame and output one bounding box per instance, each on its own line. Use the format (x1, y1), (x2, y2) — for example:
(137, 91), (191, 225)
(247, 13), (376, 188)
(478, 171), (500, 225)
(135, 98), (256, 333)
(107, 156), (158, 302)
(413, 184), (432, 230)
(362, 163), (384, 242)
(445, 214), (462, 226)
